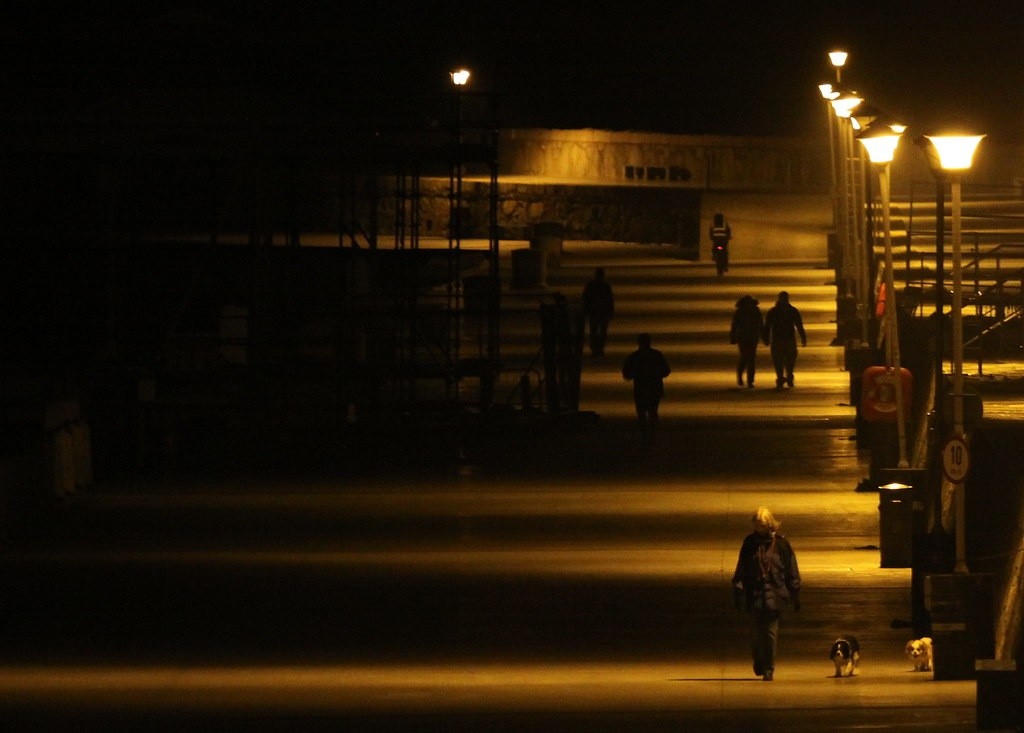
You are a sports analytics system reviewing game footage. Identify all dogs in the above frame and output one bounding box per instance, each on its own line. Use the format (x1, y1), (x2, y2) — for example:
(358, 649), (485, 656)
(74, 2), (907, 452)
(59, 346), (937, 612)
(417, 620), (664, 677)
(904, 636), (934, 671)
(830, 635), (859, 677)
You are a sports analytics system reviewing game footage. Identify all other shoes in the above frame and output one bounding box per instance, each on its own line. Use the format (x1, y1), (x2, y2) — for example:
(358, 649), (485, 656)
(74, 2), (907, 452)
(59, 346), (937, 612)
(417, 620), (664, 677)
(738, 381), (744, 385)
(787, 375), (794, 387)
(764, 671), (772, 680)
(777, 377), (786, 388)
(748, 382), (754, 388)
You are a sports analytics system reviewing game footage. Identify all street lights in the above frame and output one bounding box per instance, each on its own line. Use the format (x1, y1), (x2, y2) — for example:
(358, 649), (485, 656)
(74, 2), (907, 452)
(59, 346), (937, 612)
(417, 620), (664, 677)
(923, 126), (995, 624)
(446, 65), (504, 337)
(856, 123), (929, 493)
(813, 46), (876, 385)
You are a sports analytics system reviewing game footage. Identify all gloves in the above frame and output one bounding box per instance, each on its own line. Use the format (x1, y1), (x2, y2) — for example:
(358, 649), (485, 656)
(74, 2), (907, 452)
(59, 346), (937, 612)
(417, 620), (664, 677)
(790, 593), (800, 610)
(735, 592), (742, 612)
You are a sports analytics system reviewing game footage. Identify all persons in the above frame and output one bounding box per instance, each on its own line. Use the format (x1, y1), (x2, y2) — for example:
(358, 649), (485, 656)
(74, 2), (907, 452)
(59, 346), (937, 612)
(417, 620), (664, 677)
(544, 211), (807, 445)
(734, 508), (800, 681)
